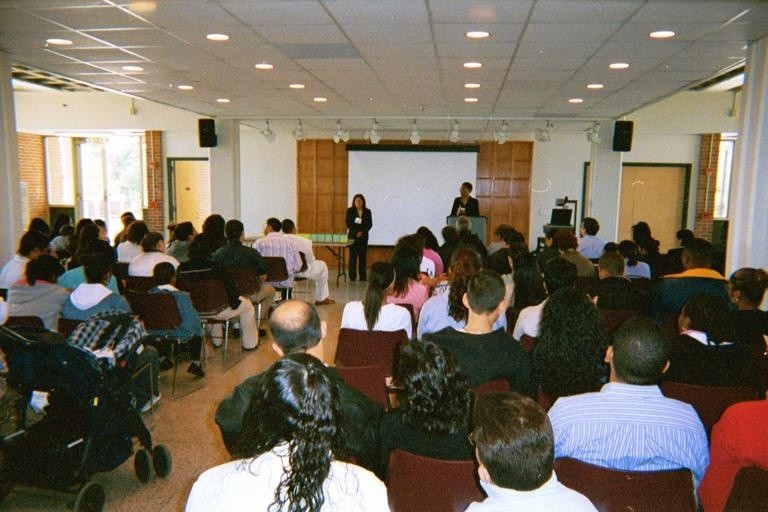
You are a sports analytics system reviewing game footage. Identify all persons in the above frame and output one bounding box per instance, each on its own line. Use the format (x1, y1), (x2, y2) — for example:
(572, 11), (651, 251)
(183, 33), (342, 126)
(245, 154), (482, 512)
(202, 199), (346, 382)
(346, 194), (373, 281)
(450, 182), (481, 216)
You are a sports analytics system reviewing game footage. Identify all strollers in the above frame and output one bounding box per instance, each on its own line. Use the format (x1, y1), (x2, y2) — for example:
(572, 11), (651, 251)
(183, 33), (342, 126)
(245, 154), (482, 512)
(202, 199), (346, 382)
(0, 301), (178, 512)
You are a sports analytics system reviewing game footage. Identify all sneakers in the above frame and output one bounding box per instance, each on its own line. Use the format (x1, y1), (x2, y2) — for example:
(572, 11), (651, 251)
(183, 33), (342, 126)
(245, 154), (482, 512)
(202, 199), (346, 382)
(161, 358), (173, 370)
(258, 328), (266, 338)
(231, 328), (240, 337)
(130, 389), (162, 412)
(187, 361), (205, 378)
(314, 297), (336, 306)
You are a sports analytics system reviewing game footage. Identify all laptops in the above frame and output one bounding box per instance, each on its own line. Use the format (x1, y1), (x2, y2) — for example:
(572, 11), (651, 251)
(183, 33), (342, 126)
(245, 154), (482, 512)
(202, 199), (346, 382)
(548, 209), (572, 225)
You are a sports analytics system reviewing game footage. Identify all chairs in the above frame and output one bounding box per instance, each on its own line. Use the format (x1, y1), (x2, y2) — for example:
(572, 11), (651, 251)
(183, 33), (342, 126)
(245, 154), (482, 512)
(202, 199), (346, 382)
(0, 237), (313, 431)
(323, 277), (762, 512)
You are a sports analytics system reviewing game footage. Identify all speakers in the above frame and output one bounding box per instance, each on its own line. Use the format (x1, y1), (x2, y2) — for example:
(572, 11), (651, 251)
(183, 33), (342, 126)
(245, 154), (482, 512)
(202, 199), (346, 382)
(199, 119), (217, 147)
(613, 121), (633, 152)
